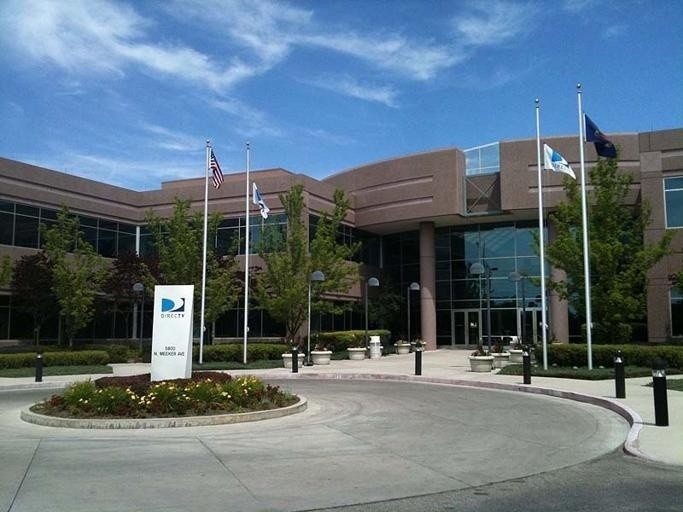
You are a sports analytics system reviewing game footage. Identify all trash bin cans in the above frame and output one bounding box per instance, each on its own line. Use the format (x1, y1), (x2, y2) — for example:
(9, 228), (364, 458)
(369, 335), (381, 358)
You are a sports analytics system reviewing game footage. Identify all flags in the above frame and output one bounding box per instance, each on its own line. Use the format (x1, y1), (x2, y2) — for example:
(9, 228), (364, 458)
(252, 182), (270, 221)
(542, 142), (577, 182)
(582, 113), (617, 158)
(208, 146), (223, 190)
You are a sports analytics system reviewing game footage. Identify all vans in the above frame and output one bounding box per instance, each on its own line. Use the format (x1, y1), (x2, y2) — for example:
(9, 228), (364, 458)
(480, 335), (520, 349)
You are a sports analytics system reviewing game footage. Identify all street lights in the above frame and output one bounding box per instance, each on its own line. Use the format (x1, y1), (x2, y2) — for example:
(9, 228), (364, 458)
(133, 282), (145, 353)
(363, 277), (380, 357)
(509, 271), (523, 350)
(406, 283), (420, 352)
(305, 271), (325, 366)
(471, 262), (485, 351)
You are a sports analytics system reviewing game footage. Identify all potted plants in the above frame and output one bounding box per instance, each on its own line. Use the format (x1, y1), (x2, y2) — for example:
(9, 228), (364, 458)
(347, 334), (365, 361)
(393, 334), (411, 354)
(490, 338), (509, 366)
(511, 337), (526, 365)
(470, 338), (493, 371)
(310, 332), (333, 366)
(283, 341), (305, 369)
